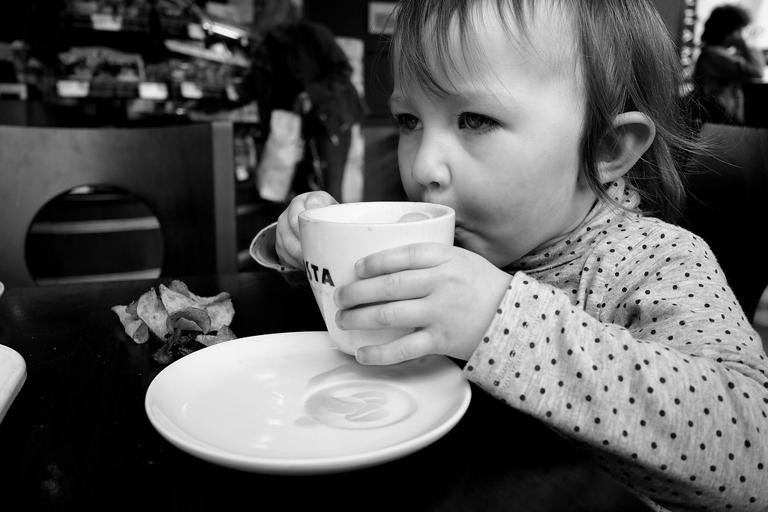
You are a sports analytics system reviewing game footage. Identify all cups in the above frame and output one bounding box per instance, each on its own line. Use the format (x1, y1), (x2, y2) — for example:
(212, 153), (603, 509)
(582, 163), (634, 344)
(298, 202), (456, 357)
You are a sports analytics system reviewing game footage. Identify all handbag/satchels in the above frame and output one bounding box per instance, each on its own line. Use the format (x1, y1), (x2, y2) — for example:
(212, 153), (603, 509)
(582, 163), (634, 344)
(258, 97), (309, 202)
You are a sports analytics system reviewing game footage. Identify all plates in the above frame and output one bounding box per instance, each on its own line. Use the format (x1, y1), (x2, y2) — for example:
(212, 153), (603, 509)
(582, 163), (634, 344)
(146, 332), (472, 473)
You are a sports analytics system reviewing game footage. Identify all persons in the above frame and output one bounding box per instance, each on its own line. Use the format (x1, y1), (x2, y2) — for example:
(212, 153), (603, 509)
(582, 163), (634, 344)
(249, 0), (768, 512)
(690, 3), (764, 128)
(252, 0), (364, 201)
